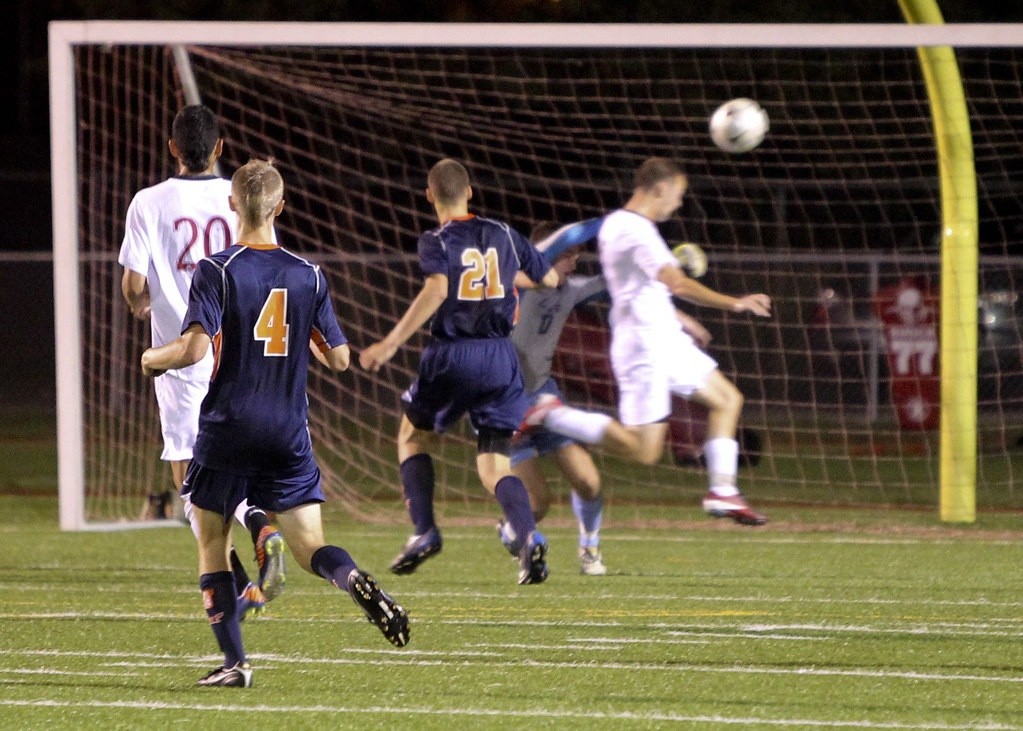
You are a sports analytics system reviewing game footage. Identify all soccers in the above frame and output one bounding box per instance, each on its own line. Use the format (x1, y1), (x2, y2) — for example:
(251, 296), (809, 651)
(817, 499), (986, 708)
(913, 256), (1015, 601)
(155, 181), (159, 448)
(707, 97), (770, 155)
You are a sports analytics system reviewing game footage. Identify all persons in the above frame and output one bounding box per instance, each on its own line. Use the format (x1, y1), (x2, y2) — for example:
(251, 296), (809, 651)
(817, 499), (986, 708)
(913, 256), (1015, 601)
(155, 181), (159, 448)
(357, 158), (560, 588)
(514, 215), (607, 580)
(139, 158), (411, 689)
(115, 107), (285, 625)
(510, 157), (774, 528)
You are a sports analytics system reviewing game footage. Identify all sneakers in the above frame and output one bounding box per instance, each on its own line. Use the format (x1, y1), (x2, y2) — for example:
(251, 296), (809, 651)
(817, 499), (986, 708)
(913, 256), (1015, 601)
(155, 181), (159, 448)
(495, 518), (524, 557)
(701, 491), (770, 525)
(390, 525), (442, 577)
(517, 530), (549, 586)
(510, 391), (563, 449)
(195, 658), (254, 688)
(577, 541), (607, 576)
(252, 524), (288, 602)
(346, 568), (411, 649)
(235, 579), (267, 624)
